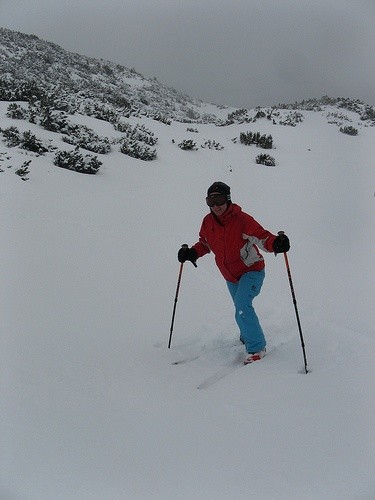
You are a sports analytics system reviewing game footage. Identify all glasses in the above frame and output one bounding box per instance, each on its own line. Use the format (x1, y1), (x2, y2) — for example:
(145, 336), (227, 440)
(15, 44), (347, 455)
(206, 193), (227, 207)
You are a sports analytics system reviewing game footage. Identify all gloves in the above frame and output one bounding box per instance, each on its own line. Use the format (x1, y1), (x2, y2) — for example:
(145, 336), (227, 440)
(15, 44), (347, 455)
(272, 235), (290, 256)
(178, 248), (198, 268)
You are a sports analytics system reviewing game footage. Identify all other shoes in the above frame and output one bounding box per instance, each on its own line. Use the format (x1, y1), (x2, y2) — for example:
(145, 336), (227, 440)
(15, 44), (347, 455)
(244, 349), (266, 365)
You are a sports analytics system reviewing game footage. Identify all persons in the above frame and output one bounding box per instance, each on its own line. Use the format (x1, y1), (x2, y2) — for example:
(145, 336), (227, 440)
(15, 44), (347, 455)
(178, 182), (290, 365)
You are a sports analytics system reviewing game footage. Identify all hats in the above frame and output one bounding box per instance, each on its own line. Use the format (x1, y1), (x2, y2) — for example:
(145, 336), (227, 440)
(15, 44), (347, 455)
(207, 182), (231, 196)
